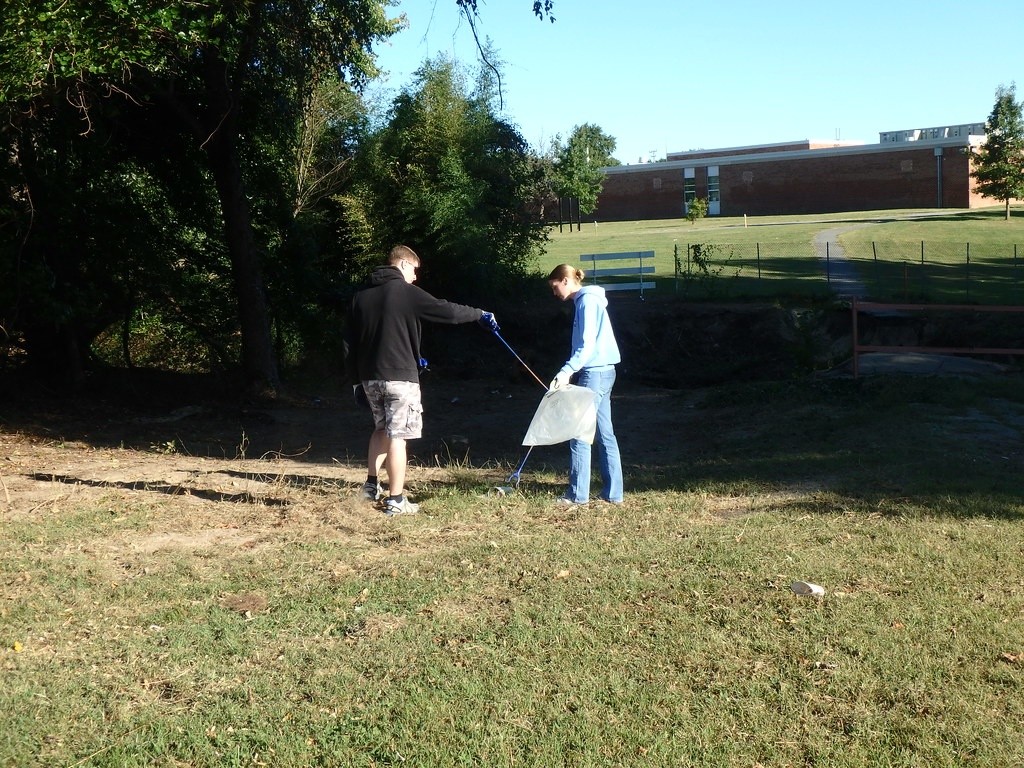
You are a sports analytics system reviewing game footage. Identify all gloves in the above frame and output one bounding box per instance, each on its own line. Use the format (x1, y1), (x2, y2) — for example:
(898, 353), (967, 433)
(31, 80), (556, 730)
(481, 310), (500, 330)
(554, 371), (569, 388)
(419, 358), (427, 368)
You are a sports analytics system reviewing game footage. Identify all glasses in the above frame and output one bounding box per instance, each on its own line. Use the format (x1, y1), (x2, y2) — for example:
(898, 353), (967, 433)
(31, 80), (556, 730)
(406, 261), (419, 275)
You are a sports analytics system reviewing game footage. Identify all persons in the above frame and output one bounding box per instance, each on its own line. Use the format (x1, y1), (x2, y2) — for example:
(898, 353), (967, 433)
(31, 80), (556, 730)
(348, 245), (502, 517)
(548, 263), (625, 507)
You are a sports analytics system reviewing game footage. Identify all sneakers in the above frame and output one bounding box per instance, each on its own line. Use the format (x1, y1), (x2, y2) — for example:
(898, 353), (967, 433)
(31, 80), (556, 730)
(385, 496), (419, 516)
(556, 497), (589, 505)
(364, 481), (382, 500)
